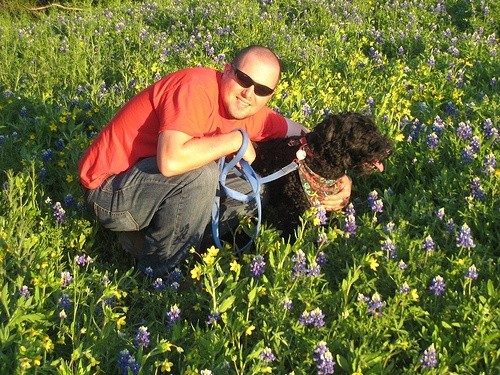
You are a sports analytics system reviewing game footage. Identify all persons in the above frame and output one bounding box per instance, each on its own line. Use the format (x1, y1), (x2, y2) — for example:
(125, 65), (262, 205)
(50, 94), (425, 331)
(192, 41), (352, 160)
(77, 45), (352, 288)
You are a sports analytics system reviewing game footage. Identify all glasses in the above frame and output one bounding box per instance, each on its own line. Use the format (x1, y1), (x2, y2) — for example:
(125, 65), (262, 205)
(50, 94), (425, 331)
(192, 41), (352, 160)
(231, 63), (276, 96)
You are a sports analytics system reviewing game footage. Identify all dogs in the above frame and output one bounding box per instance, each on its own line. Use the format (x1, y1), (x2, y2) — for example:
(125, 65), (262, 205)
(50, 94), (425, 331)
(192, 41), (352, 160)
(216, 110), (393, 255)
